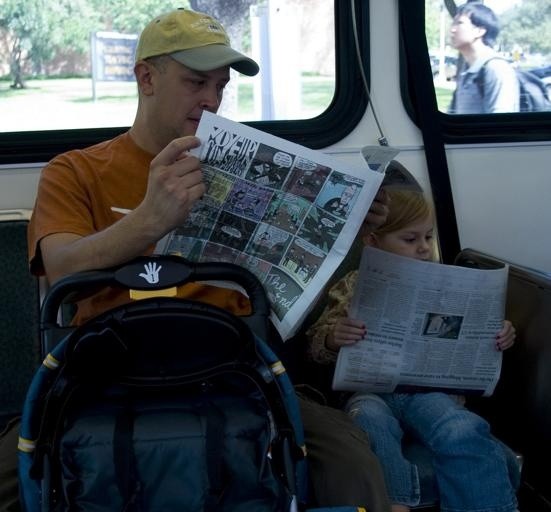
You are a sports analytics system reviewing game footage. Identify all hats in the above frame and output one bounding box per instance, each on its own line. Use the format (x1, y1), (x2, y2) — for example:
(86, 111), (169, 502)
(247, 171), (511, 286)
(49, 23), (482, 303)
(134, 7), (260, 76)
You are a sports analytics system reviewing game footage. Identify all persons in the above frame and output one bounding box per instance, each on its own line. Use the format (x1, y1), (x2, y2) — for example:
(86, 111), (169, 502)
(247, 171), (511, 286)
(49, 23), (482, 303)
(26, 6), (392, 338)
(308, 182), (525, 510)
(447, 2), (524, 114)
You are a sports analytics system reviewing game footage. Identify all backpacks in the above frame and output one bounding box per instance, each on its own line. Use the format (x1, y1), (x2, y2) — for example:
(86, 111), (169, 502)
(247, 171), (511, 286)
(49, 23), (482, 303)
(477, 57), (549, 112)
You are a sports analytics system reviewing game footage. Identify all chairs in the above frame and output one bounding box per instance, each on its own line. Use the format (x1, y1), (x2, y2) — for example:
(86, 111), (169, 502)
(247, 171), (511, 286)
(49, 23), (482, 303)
(1, 207), (528, 512)
